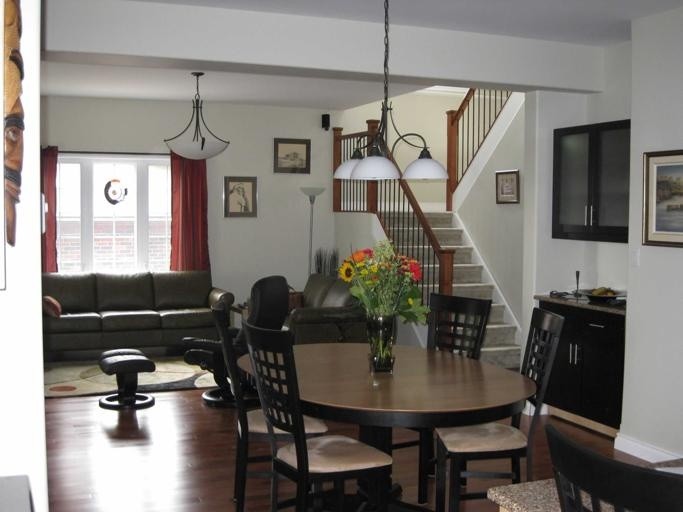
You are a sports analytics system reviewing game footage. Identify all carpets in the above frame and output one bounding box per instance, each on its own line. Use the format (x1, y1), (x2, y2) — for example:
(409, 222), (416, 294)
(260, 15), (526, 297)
(43, 357), (235, 399)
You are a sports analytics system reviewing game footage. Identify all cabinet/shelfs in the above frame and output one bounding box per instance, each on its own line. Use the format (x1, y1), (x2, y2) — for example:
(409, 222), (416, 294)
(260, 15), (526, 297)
(549, 118), (633, 245)
(531, 290), (628, 447)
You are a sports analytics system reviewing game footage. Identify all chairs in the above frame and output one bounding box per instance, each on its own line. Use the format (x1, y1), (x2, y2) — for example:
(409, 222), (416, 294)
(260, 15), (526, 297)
(185, 274), (291, 405)
(238, 323), (397, 512)
(429, 307), (567, 510)
(539, 423), (682, 512)
(204, 308), (329, 511)
(362, 291), (492, 504)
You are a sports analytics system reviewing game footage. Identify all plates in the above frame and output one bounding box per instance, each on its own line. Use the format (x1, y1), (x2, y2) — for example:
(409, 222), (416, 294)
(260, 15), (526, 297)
(573, 287), (627, 301)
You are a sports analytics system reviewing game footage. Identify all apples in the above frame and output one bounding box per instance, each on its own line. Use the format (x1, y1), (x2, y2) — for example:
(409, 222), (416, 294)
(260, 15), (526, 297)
(592, 287), (614, 296)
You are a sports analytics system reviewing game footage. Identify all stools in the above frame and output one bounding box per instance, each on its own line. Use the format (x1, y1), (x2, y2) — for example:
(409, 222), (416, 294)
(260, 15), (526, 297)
(94, 348), (157, 412)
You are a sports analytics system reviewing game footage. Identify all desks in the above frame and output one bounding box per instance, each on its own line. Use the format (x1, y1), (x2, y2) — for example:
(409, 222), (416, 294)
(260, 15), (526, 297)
(487, 452), (682, 511)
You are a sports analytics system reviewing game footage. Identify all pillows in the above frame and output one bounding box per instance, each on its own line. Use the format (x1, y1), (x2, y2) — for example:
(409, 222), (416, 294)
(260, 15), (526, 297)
(41, 292), (62, 319)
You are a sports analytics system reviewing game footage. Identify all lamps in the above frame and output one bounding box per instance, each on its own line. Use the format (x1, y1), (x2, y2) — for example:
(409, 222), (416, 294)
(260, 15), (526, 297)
(300, 186), (323, 280)
(329, 0), (452, 181)
(162, 69), (231, 162)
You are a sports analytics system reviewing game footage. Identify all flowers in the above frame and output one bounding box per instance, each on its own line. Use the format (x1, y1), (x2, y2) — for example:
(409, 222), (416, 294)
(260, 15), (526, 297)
(338, 241), (431, 368)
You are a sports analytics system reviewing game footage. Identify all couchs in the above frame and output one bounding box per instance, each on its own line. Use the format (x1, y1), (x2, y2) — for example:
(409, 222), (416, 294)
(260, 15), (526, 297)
(40, 270), (236, 362)
(283, 271), (370, 343)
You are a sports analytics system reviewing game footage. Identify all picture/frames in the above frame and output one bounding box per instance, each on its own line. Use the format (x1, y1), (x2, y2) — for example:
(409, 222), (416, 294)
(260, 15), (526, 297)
(273, 136), (312, 173)
(642, 147), (683, 250)
(222, 174), (257, 221)
(492, 168), (519, 204)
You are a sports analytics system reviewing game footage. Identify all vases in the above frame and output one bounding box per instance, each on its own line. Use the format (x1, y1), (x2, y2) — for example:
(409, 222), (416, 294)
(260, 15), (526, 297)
(362, 308), (396, 376)
